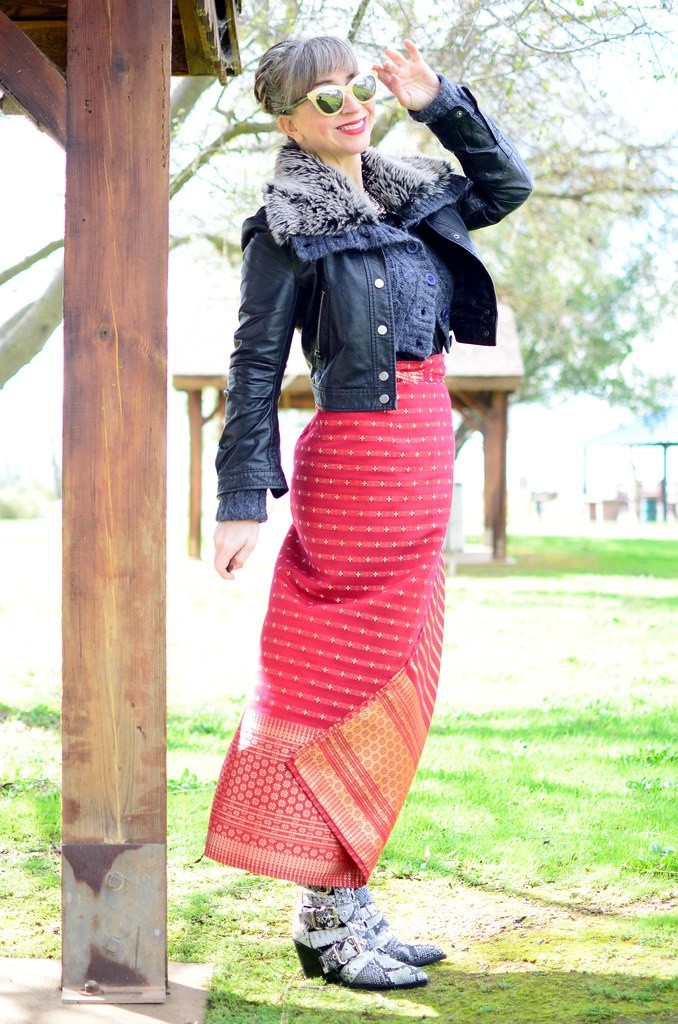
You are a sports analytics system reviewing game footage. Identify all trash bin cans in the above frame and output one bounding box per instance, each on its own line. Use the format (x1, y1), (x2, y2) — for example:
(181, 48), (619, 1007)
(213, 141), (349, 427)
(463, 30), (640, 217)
(644, 497), (657, 521)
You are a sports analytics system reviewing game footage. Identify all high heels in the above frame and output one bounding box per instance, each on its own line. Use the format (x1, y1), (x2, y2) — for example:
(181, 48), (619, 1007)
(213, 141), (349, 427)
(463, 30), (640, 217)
(294, 886), (429, 991)
(353, 884), (446, 965)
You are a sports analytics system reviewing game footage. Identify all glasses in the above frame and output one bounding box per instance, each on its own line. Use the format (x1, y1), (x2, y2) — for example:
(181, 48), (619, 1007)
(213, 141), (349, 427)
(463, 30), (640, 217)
(282, 72), (377, 118)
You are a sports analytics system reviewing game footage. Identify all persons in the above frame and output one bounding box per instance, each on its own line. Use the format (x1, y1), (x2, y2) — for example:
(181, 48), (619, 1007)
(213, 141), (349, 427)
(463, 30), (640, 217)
(203, 30), (534, 990)
